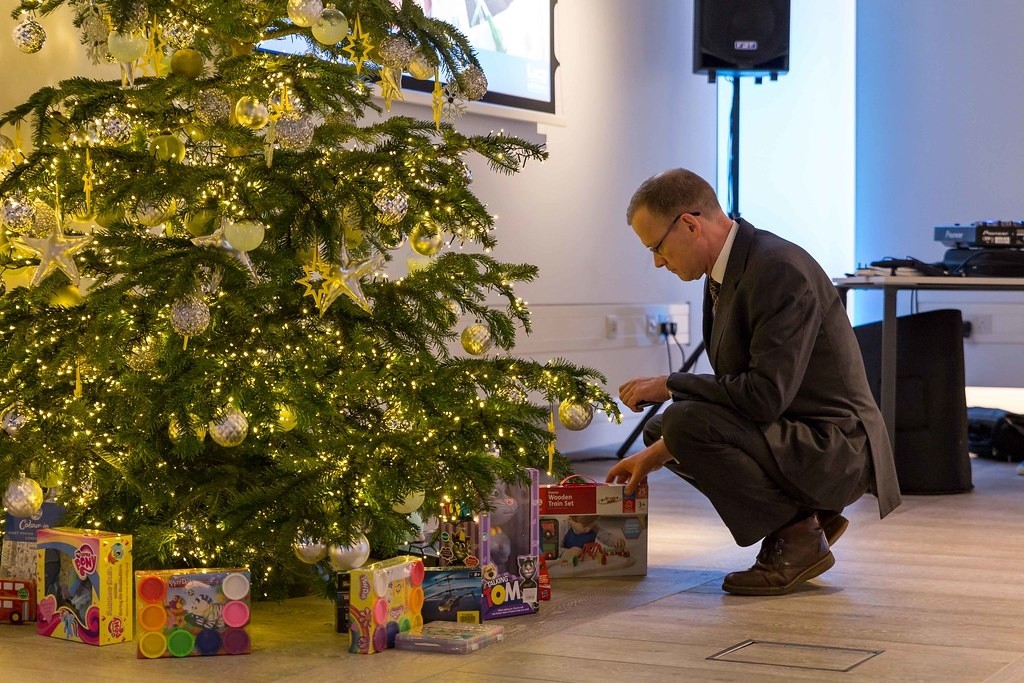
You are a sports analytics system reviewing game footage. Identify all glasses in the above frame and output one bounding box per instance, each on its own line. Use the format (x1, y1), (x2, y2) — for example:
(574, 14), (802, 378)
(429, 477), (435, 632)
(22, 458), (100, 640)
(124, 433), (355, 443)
(647, 212), (701, 256)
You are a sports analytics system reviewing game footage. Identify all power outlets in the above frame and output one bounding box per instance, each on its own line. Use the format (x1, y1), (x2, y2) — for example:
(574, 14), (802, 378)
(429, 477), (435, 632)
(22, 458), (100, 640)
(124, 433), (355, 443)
(645, 312), (686, 335)
(606, 313), (621, 339)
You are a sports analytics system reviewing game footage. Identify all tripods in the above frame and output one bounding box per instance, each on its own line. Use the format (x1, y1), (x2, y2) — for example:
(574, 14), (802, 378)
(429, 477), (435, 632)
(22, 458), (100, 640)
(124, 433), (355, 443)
(614, 79), (741, 457)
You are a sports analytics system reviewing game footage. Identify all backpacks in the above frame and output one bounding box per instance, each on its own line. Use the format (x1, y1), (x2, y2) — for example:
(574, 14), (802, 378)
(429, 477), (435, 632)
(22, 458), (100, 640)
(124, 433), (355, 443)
(967, 405), (1024, 461)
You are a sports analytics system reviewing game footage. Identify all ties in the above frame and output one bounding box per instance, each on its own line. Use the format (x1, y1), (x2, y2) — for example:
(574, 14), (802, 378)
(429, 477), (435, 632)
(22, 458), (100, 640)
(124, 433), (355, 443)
(707, 276), (720, 322)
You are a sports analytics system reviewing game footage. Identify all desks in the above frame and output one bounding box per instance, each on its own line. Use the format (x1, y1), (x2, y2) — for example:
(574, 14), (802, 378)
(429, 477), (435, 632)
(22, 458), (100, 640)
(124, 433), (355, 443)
(831, 272), (1024, 460)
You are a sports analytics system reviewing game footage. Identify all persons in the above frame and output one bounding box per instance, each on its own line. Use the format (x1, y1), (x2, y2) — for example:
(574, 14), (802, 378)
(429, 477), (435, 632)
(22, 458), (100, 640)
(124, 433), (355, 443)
(604, 167), (903, 596)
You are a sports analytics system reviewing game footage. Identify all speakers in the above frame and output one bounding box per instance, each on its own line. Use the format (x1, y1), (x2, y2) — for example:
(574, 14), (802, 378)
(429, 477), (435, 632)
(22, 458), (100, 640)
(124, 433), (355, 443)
(692, 0), (790, 84)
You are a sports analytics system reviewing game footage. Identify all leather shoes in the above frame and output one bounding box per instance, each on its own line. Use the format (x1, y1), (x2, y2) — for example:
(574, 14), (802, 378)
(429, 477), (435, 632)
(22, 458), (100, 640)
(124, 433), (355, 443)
(723, 510), (854, 595)
